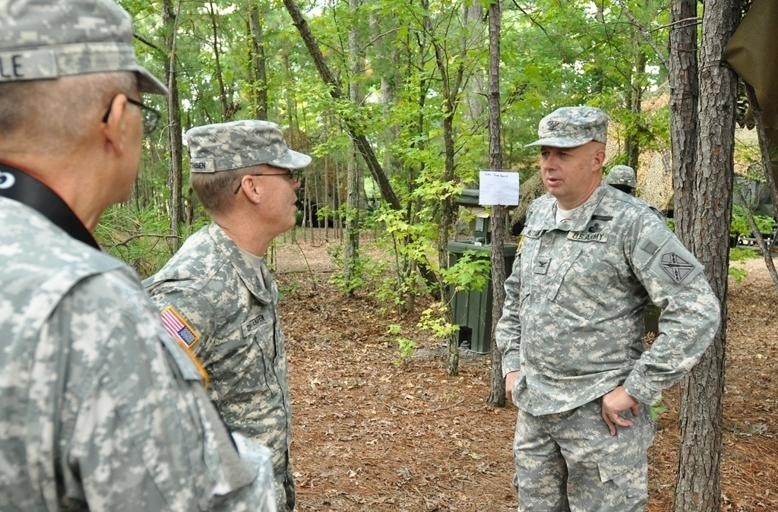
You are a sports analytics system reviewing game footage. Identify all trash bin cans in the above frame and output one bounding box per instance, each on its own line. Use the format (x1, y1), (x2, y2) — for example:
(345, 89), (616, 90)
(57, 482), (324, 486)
(447, 188), (518, 353)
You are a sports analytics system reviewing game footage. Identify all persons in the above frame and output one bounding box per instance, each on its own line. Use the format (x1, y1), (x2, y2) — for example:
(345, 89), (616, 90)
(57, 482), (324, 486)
(494, 105), (724, 512)
(148, 118), (314, 512)
(0, 0), (268, 512)
(605, 165), (638, 198)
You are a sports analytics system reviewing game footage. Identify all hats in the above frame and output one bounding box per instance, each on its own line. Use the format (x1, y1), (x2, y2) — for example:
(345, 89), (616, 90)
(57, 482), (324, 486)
(604, 163), (639, 189)
(524, 106), (609, 150)
(0, 0), (171, 98)
(184, 119), (313, 173)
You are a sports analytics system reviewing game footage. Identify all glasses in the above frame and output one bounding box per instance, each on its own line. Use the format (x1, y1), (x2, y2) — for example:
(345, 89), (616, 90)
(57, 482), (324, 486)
(101, 99), (162, 135)
(232, 169), (300, 195)
